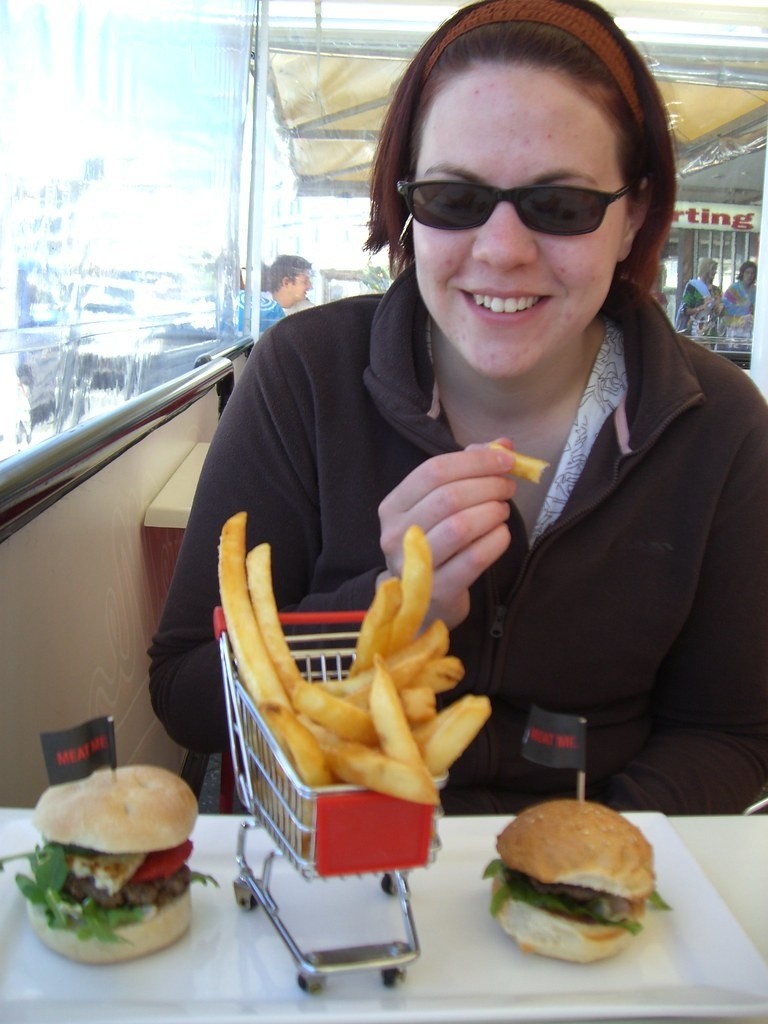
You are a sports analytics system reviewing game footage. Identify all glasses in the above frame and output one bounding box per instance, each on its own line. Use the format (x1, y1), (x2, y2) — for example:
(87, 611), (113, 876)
(395, 175), (642, 238)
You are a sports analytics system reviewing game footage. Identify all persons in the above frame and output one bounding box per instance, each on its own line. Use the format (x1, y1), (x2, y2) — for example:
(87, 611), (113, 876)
(220, 263), (286, 333)
(269, 254), (314, 317)
(722, 261), (757, 338)
(649, 258), (724, 335)
(148, 0), (768, 815)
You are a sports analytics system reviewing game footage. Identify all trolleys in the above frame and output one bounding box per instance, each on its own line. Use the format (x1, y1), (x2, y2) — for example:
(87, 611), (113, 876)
(210, 603), (424, 997)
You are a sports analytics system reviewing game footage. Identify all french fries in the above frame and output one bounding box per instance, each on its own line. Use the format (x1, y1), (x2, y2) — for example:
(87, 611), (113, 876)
(487, 443), (550, 484)
(218, 512), (490, 852)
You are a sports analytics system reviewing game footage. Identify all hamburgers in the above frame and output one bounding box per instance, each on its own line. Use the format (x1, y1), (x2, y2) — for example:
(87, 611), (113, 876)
(0, 763), (222, 964)
(484, 798), (672, 967)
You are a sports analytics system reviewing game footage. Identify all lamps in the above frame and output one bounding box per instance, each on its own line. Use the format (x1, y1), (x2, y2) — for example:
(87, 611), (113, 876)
(267, 0), (768, 54)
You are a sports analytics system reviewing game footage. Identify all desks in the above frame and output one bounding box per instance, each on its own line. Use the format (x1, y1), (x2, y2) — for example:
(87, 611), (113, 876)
(145, 442), (210, 529)
(0, 814), (768, 1024)
